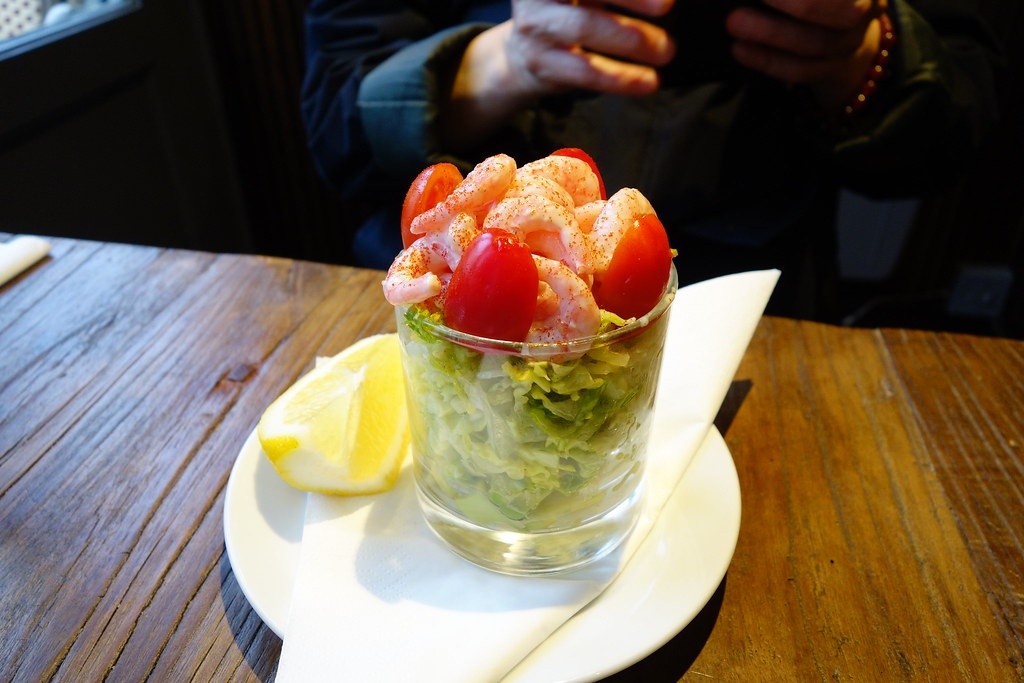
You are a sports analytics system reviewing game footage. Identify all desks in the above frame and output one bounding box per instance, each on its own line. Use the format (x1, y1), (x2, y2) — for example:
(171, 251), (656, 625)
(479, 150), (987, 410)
(0, 229), (1024, 682)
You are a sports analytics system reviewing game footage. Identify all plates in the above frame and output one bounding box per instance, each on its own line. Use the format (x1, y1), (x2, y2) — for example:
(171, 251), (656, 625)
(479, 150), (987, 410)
(221, 332), (744, 683)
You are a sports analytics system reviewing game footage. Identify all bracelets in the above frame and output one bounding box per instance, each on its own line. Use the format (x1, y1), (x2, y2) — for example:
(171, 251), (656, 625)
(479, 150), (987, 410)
(843, 13), (896, 115)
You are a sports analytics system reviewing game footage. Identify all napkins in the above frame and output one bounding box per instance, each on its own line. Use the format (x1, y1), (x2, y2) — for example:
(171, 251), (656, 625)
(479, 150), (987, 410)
(272, 265), (781, 683)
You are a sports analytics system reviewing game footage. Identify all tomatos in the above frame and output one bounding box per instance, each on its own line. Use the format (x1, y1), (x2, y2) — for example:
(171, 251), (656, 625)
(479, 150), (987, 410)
(401, 147), (673, 354)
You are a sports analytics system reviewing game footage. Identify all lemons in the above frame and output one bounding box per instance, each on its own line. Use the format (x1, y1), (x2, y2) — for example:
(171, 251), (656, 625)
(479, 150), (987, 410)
(256, 330), (409, 498)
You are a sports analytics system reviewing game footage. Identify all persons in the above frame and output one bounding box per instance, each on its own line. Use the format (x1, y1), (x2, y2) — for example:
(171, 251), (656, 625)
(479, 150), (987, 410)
(300, 0), (1006, 334)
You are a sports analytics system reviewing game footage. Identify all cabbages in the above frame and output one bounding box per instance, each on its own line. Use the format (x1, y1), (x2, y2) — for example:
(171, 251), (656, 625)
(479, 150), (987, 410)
(400, 303), (668, 535)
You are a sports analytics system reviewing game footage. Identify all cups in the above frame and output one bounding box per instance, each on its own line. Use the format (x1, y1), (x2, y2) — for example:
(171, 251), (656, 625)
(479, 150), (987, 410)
(394, 260), (679, 579)
